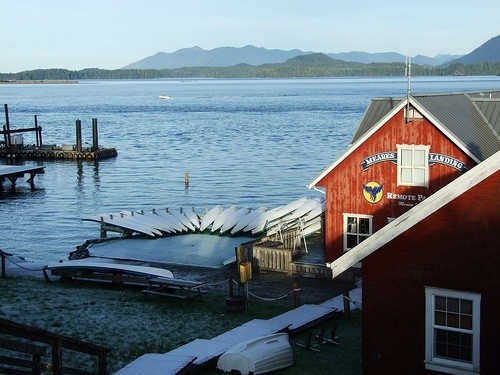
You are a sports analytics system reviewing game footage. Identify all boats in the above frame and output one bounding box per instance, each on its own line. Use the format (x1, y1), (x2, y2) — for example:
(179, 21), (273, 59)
(217, 330), (296, 375)
(159, 96), (171, 100)
(50, 259), (174, 282)
(82, 197), (327, 240)
